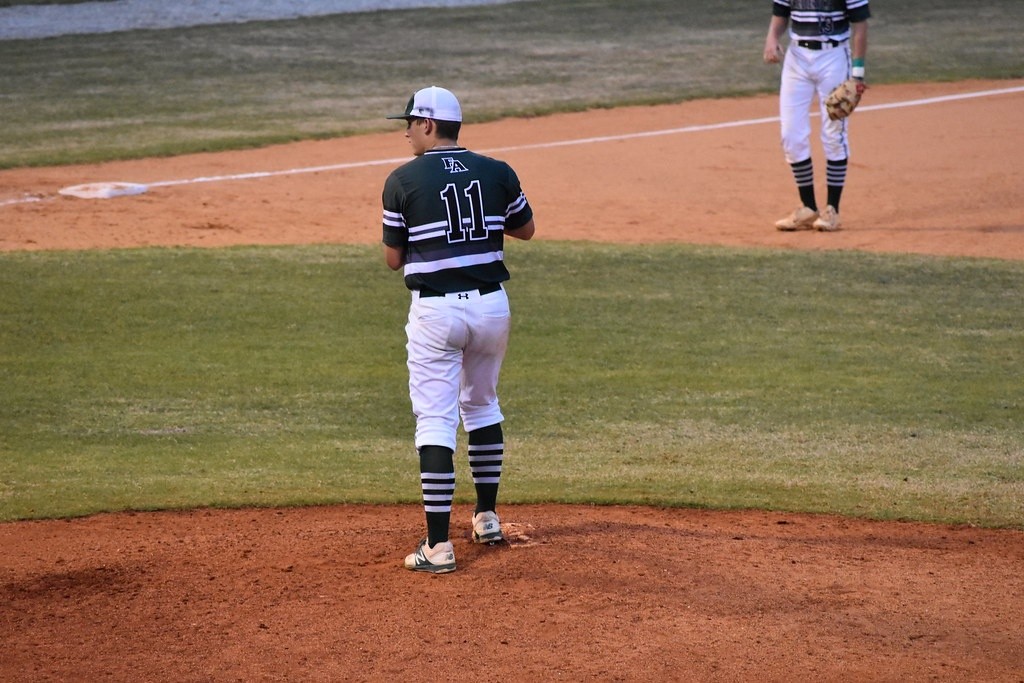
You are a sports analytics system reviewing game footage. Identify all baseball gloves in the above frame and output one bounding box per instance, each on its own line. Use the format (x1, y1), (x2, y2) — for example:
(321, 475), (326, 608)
(826, 80), (868, 121)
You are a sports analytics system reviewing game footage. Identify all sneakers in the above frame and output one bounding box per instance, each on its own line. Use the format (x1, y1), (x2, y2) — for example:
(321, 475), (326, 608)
(813, 205), (840, 231)
(775, 207), (819, 230)
(472, 510), (503, 544)
(405, 537), (456, 573)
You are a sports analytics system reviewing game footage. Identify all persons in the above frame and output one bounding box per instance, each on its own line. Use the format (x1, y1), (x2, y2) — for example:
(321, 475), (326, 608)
(763, 0), (873, 231)
(382, 86), (536, 575)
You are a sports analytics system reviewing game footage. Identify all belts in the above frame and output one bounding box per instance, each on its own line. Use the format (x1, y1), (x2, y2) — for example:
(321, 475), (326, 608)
(420, 283), (501, 297)
(799, 40), (847, 50)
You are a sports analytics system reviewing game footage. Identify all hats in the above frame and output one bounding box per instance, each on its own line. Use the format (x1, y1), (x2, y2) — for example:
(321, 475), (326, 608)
(385, 84), (462, 122)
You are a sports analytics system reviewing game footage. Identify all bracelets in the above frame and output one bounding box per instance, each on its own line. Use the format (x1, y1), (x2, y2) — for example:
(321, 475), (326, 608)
(849, 57), (868, 80)
